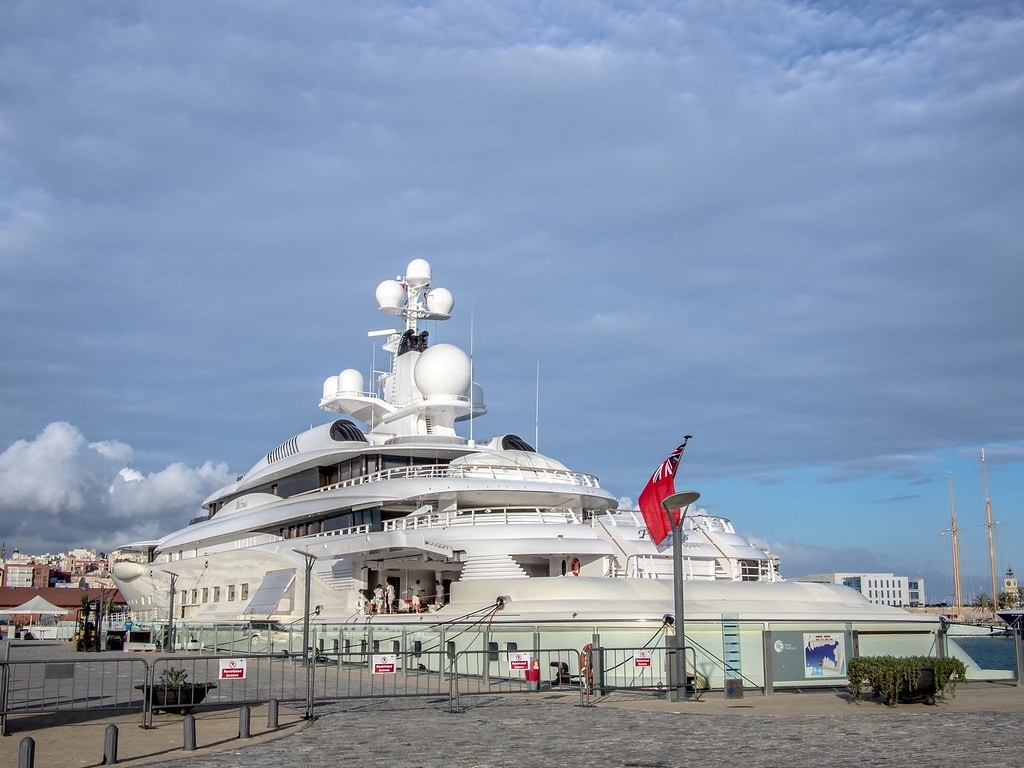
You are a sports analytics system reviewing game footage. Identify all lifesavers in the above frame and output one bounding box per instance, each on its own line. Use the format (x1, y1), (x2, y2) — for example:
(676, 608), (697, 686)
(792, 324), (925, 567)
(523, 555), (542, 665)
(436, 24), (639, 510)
(580, 643), (593, 677)
(571, 557), (580, 576)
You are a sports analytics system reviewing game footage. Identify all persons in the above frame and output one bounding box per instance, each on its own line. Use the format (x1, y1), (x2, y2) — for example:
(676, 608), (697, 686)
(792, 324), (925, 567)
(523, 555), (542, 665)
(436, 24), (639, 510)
(409, 579), (426, 613)
(124, 617), (136, 632)
(434, 580), (445, 610)
(374, 584), (386, 613)
(358, 589), (376, 613)
(385, 580), (396, 613)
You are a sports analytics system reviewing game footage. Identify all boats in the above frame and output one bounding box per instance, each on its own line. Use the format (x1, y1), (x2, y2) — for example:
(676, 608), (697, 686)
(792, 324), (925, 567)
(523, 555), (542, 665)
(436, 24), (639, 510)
(994, 610), (1024, 637)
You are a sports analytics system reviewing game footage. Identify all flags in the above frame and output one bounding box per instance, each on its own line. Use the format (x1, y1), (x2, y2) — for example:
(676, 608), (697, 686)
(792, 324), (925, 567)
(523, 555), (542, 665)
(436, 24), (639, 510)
(638, 443), (686, 545)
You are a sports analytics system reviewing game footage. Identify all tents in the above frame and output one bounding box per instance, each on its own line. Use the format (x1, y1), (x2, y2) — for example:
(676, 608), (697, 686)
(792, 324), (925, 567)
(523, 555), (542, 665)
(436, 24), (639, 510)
(0, 595), (68, 627)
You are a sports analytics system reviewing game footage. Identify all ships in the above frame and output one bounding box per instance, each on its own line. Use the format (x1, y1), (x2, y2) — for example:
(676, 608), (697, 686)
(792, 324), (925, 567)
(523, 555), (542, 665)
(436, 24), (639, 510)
(101, 258), (1016, 689)
